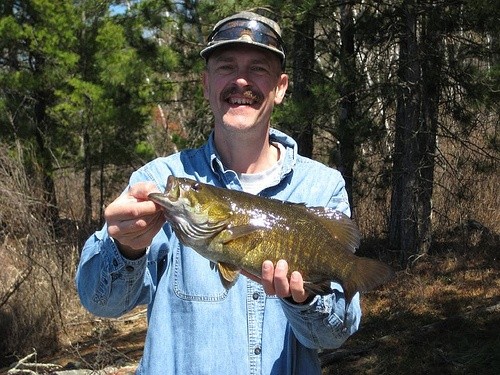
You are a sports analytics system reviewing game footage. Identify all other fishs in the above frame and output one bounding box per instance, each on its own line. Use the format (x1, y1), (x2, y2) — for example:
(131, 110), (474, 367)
(148, 175), (395, 305)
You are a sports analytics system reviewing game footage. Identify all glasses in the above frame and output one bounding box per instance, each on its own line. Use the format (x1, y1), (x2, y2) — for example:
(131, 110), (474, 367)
(207, 18), (285, 52)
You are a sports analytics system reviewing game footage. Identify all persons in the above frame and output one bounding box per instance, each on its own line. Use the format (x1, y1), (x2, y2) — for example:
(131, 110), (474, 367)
(77, 9), (362, 374)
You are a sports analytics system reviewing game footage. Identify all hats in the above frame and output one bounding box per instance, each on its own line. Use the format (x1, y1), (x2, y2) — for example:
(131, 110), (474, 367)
(200, 11), (285, 64)
